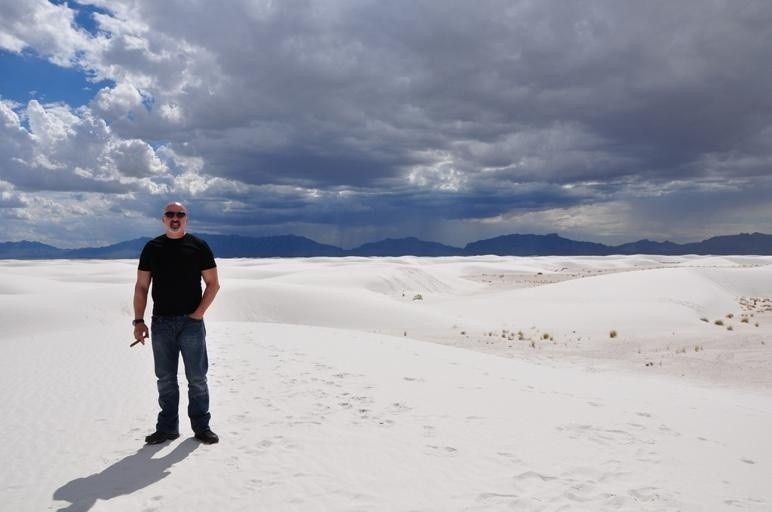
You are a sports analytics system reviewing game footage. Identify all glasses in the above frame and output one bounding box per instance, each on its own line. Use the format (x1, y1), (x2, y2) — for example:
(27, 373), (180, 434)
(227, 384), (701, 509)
(165, 211), (186, 218)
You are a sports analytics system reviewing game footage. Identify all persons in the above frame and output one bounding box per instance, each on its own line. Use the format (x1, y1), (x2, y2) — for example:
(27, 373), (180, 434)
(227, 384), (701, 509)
(132, 200), (223, 445)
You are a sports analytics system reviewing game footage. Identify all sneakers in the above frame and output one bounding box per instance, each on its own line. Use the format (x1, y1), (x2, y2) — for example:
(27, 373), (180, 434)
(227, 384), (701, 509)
(195, 429), (218, 443)
(145, 431), (180, 445)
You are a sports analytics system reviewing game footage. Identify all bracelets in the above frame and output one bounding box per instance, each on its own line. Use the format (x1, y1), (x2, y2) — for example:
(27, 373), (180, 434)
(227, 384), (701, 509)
(132, 319), (144, 326)
